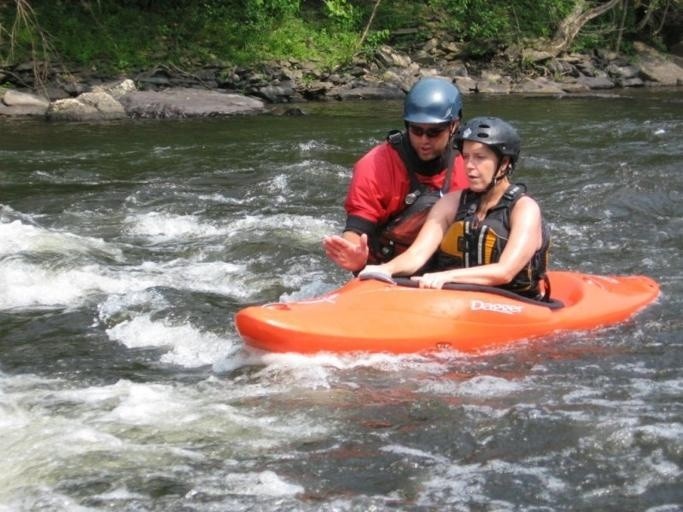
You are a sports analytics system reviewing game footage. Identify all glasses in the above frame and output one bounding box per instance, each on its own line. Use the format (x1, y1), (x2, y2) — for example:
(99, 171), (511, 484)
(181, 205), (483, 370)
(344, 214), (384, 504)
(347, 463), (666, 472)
(408, 123), (450, 139)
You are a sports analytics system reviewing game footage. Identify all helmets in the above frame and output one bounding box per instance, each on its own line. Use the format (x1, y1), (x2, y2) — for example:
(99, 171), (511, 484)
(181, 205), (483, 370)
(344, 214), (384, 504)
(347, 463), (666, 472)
(402, 77), (463, 127)
(453, 115), (522, 167)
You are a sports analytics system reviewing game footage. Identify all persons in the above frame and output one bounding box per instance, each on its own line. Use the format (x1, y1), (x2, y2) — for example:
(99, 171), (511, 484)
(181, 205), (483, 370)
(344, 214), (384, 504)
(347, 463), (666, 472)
(321, 77), (470, 278)
(358, 115), (552, 303)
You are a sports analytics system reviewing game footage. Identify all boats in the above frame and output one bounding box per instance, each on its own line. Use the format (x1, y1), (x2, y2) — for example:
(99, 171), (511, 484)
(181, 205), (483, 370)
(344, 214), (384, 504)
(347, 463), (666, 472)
(234, 271), (658, 351)
